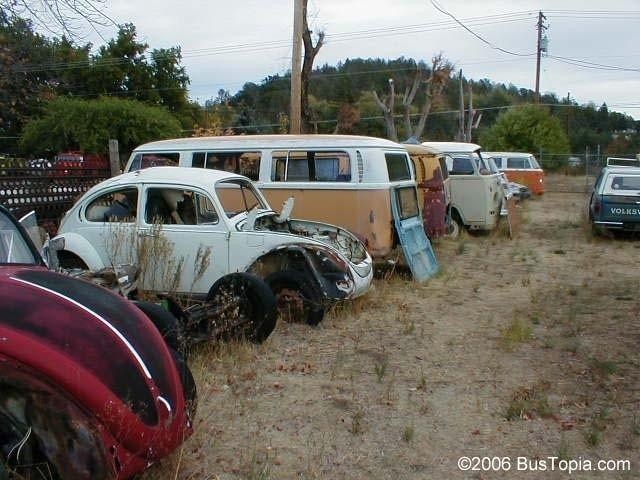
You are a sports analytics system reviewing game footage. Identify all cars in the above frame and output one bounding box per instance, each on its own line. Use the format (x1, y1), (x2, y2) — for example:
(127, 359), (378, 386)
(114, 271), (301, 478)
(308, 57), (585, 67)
(45, 162), (386, 334)
(586, 157), (640, 236)
(0, 200), (214, 478)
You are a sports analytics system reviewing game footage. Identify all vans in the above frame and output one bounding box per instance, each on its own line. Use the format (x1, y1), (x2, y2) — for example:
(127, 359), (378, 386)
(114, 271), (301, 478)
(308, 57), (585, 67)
(26, 134), (550, 256)
